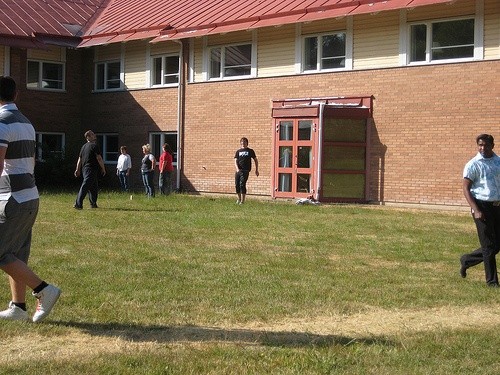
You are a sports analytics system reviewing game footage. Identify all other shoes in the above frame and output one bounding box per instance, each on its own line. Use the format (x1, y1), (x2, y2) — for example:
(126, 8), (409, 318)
(460, 256), (467, 278)
(73, 205), (83, 210)
(236, 200), (244, 205)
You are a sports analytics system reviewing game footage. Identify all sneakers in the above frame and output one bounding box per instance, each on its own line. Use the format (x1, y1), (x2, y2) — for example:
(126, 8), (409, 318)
(31, 284), (62, 323)
(0, 301), (29, 319)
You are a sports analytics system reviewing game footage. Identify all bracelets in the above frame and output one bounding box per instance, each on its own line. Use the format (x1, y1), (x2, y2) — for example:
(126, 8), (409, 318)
(152, 167), (154, 170)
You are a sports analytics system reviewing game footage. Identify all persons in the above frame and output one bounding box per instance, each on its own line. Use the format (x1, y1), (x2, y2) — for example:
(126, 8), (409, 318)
(116, 146), (132, 194)
(159, 144), (173, 194)
(460, 134), (500, 287)
(0, 78), (62, 324)
(141, 144), (156, 198)
(234, 137), (259, 205)
(73, 130), (106, 210)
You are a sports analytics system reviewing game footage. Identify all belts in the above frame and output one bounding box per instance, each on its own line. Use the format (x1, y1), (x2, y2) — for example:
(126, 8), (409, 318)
(476, 200), (500, 206)
